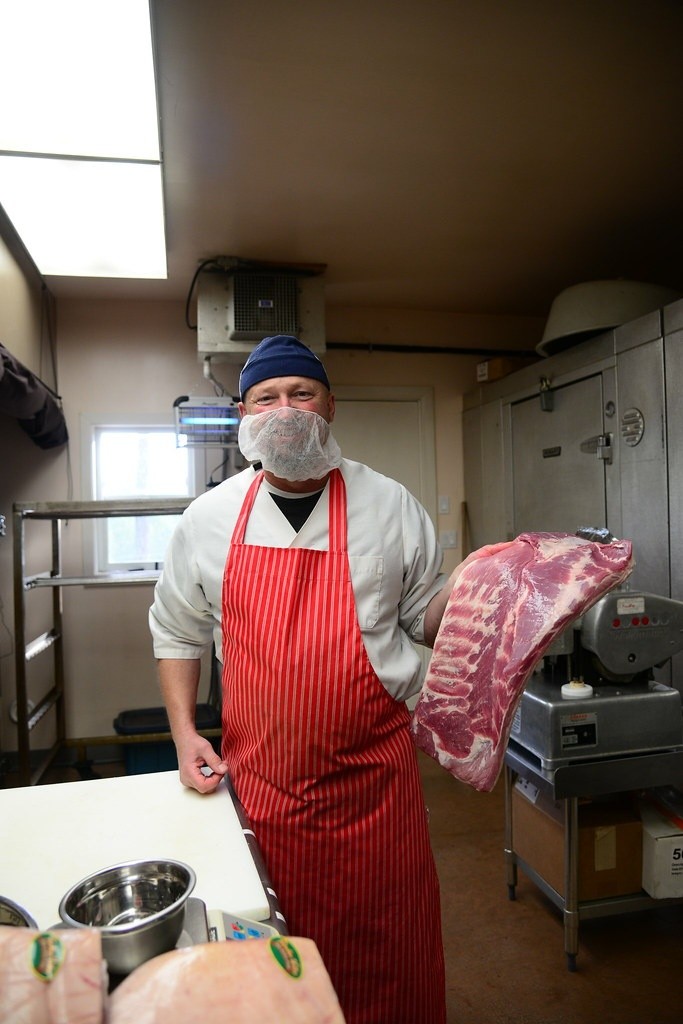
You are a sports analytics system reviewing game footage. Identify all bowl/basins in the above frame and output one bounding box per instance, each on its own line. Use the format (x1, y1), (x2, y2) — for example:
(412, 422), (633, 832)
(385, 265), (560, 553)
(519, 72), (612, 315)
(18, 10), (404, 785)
(0, 896), (38, 928)
(57, 858), (195, 974)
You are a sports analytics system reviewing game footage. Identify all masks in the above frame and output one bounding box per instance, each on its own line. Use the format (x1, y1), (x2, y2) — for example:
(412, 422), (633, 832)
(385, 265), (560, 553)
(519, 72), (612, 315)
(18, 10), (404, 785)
(238, 406), (342, 482)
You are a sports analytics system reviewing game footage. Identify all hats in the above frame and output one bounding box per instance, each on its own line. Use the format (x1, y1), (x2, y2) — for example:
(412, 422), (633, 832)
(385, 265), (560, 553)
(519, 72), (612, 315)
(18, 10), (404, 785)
(238, 335), (331, 403)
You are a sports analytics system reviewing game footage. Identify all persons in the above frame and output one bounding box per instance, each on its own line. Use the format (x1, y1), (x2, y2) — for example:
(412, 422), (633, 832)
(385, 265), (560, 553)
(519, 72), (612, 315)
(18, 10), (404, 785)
(146, 334), (520, 1024)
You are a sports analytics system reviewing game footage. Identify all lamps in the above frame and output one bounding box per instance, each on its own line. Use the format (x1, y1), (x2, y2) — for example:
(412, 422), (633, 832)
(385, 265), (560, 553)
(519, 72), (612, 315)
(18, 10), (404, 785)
(179, 416), (241, 426)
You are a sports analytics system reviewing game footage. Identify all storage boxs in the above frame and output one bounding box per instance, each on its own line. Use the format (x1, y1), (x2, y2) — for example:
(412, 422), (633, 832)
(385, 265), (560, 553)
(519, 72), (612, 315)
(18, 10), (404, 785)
(476, 356), (538, 382)
(113, 704), (222, 775)
(511, 776), (683, 903)
